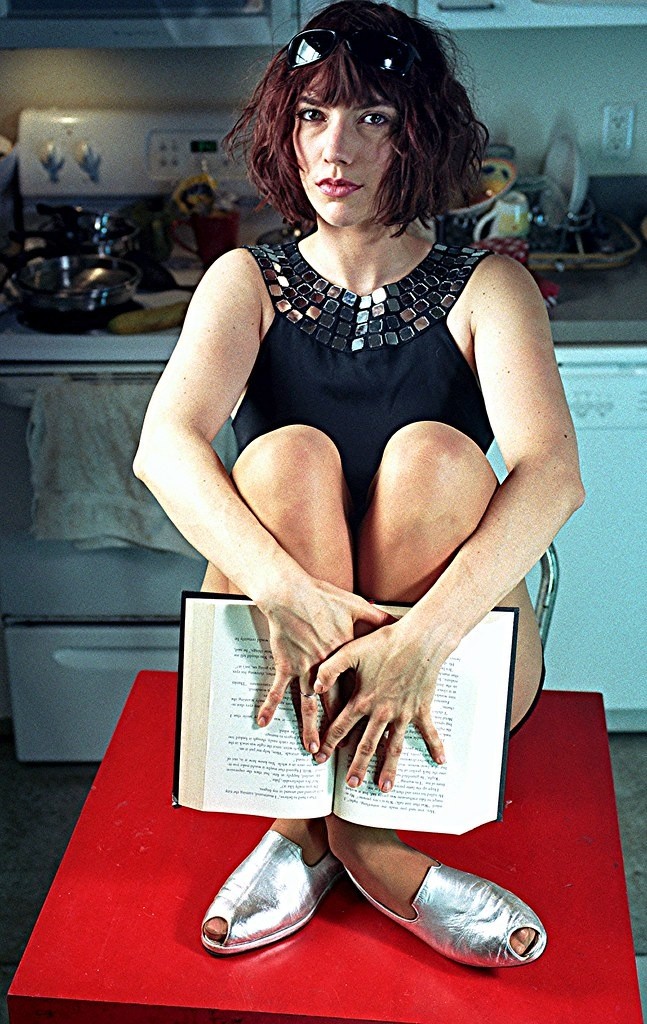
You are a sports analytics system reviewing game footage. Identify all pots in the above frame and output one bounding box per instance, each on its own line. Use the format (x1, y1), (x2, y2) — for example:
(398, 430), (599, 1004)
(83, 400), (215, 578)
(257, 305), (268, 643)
(7, 206), (140, 261)
(11, 254), (142, 315)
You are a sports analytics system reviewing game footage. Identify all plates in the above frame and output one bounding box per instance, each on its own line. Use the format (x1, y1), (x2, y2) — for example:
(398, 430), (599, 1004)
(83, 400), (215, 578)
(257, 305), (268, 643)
(541, 135), (588, 226)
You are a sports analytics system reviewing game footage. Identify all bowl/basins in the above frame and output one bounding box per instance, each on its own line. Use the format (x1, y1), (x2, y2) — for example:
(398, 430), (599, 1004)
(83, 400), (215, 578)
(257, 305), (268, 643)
(446, 156), (516, 216)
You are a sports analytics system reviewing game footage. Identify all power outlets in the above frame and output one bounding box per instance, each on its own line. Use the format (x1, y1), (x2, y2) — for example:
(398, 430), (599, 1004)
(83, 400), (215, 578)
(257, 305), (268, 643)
(599, 100), (635, 161)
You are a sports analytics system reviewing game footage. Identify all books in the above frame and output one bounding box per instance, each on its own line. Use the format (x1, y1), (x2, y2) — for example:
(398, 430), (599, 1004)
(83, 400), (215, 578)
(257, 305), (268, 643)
(174, 589), (517, 835)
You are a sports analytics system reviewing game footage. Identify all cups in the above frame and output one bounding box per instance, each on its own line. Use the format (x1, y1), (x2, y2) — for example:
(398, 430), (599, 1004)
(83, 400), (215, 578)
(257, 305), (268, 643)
(170, 211), (238, 268)
(473, 189), (531, 241)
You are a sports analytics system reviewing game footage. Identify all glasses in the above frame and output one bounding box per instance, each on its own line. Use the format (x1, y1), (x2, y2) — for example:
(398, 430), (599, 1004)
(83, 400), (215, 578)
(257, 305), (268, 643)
(272, 28), (437, 80)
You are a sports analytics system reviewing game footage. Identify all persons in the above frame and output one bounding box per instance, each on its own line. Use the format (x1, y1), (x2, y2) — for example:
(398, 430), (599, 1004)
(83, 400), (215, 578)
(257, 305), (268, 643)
(134, 1), (587, 968)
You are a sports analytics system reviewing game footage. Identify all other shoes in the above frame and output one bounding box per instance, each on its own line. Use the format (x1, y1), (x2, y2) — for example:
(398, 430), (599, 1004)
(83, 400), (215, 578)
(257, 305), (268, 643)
(340, 858), (546, 967)
(198, 829), (343, 955)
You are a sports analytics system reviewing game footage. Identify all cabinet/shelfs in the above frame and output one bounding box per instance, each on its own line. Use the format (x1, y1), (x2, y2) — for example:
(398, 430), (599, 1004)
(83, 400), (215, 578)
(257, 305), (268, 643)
(486, 345), (647, 732)
